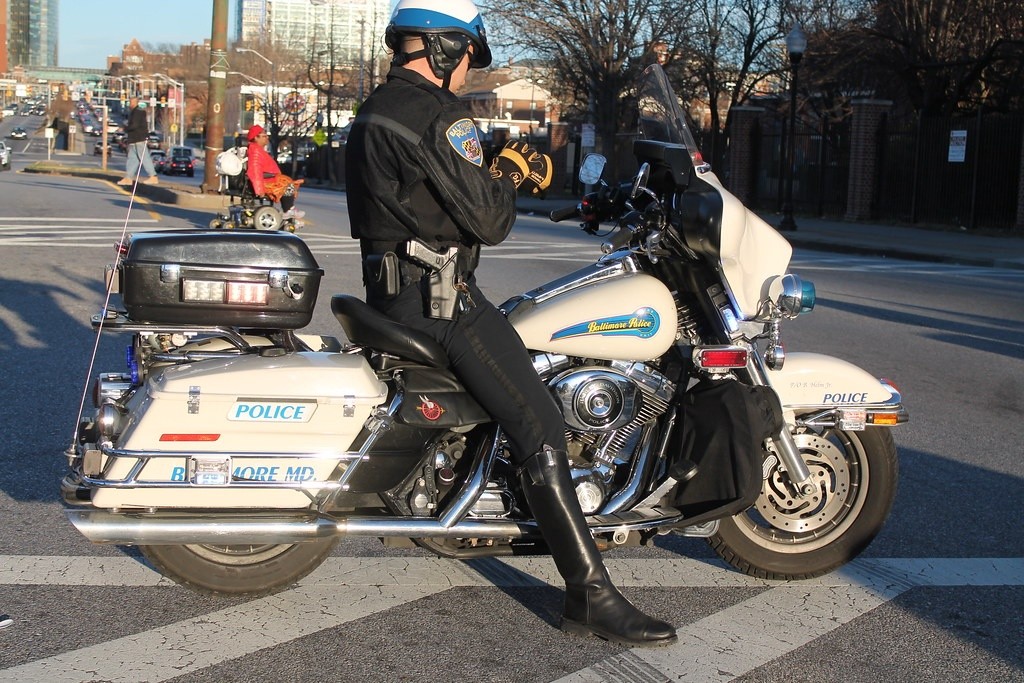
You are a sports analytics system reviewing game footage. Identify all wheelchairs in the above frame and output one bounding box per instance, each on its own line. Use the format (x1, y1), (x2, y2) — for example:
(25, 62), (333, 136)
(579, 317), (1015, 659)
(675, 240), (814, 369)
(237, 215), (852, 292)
(207, 167), (298, 236)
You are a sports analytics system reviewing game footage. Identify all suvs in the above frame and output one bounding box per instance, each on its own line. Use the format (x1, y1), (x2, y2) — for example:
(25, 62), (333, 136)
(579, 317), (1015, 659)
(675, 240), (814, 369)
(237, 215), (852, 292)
(0, 141), (12, 170)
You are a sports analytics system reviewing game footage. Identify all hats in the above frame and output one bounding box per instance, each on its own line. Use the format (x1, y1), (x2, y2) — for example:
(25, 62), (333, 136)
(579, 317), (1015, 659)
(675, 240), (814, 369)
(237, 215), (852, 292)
(247, 125), (263, 141)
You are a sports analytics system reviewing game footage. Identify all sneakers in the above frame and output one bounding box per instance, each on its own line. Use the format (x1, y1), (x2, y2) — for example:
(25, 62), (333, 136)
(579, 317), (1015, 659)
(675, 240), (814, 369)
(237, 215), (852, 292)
(282, 207), (306, 219)
(284, 220), (304, 231)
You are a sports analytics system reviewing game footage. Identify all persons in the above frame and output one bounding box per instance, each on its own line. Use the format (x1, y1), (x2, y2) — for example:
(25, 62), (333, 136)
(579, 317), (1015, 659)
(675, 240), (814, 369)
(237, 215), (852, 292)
(117, 97), (159, 185)
(234, 134), (242, 155)
(333, 0), (678, 648)
(248, 125), (305, 220)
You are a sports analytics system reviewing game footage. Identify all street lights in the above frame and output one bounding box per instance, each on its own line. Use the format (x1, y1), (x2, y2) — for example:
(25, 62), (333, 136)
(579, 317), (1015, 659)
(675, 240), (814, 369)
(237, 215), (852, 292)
(235, 47), (281, 137)
(96, 73), (185, 147)
(226, 70), (269, 133)
(309, 0), (365, 107)
(775, 22), (807, 232)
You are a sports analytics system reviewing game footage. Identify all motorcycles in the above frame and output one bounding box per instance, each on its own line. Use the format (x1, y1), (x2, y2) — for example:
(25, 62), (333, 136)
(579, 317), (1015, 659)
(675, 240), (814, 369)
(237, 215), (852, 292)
(58, 64), (911, 599)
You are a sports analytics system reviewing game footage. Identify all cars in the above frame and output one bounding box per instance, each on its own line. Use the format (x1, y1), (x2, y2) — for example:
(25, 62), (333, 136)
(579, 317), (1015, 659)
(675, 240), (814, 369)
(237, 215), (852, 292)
(2, 96), (49, 117)
(163, 156), (194, 177)
(155, 158), (167, 173)
(11, 126), (27, 140)
(146, 136), (162, 150)
(92, 141), (113, 158)
(276, 152), (304, 164)
(70, 101), (129, 150)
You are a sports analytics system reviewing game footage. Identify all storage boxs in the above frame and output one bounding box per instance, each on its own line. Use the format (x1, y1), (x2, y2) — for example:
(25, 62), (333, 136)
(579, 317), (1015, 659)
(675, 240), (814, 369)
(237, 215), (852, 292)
(104, 228), (323, 330)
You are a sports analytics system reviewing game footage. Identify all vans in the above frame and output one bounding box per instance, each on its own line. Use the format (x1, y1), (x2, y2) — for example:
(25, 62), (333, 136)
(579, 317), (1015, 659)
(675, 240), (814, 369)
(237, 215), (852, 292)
(150, 150), (166, 161)
(170, 148), (196, 166)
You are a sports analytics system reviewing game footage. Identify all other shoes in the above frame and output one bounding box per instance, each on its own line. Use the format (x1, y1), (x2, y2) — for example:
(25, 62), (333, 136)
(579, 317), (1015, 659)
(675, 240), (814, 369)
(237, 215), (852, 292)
(118, 178), (133, 186)
(143, 175), (158, 185)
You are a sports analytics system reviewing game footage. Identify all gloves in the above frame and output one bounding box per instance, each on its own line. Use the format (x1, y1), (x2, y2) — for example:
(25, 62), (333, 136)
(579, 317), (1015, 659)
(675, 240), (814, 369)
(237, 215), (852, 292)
(489, 140), (551, 194)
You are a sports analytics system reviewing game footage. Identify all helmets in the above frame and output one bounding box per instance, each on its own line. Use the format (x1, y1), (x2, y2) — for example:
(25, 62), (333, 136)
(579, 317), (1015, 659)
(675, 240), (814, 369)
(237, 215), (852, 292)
(385, 0), (492, 68)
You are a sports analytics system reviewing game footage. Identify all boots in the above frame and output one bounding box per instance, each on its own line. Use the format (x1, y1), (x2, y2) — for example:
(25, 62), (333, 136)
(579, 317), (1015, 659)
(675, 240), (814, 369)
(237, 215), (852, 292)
(517, 448), (678, 647)
